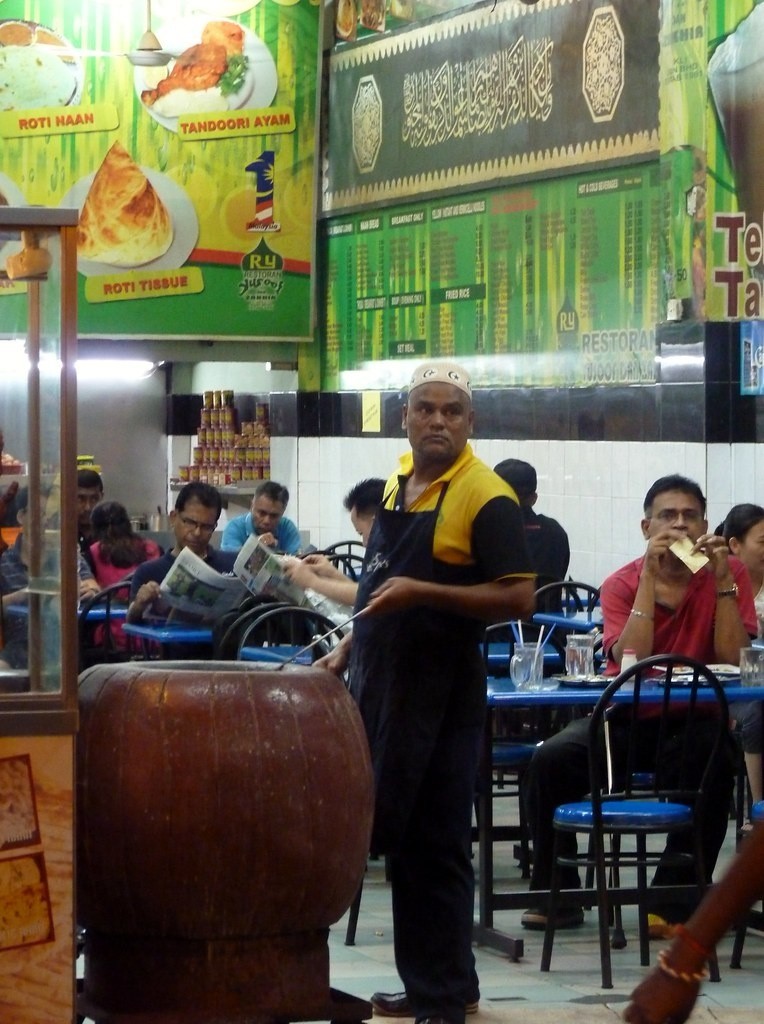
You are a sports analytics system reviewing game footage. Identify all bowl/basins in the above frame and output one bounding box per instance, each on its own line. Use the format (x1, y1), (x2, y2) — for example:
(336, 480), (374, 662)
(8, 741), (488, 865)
(129, 511), (170, 532)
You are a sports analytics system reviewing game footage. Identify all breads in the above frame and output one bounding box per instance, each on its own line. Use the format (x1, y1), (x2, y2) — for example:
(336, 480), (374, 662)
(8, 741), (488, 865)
(77, 140), (174, 268)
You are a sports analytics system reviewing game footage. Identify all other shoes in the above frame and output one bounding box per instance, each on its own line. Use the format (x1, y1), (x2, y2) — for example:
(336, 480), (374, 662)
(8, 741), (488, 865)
(522, 901), (585, 928)
(370, 992), (480, 1016)
(415, 1011), (451, 1024)
(646, 910), (674, 939)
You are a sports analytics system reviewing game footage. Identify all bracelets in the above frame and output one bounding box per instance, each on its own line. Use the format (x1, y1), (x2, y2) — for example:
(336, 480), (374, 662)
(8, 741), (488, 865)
(631, 609), (654, 622)
(656, 945), (710, 982)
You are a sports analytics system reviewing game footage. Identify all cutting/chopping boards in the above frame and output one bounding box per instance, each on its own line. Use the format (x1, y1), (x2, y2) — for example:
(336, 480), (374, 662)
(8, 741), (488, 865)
(336, 0), (415, 42)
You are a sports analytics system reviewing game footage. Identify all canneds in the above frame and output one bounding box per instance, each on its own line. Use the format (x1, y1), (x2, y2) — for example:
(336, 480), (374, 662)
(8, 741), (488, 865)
(179, 390), (271, 488)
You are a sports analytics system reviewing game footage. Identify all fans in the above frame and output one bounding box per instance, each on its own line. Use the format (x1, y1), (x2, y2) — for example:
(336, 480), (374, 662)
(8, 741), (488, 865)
(34, 5), (188, 66)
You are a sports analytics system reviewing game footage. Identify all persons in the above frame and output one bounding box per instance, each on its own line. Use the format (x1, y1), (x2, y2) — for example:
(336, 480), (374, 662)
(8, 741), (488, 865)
(521, 474), (758, 938)
(312, 361), (539, 1024)
(87, 499), (163, 654)
(284, 477), (389, 607)
(47, 469), (105, 531)
(622, 820), (764, 1024)
(125, 481), (251, 625)
(220, 480), (301, 561)
(493, 458), (569, 616)
(0, 484), (102, 669)
(715, 503), (764, 820)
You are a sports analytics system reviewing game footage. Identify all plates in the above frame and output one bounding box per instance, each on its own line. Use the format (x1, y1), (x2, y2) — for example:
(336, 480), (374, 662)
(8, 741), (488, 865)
(0, 18), (86, 108)
(336, 0), (354, 38)
(133, 16), (278, 134)
(551, 662), (740, 689)
(50, 166), (200, 277)
(0, 169), (31, 272)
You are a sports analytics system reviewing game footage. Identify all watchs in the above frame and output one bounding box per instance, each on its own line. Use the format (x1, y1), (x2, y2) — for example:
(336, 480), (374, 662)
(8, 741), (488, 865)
(718, 583), (739, 597)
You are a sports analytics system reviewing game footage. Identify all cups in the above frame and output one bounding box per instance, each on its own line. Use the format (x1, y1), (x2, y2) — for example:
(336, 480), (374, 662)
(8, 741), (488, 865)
(179, 390), (270, 489)
(739, 647), (764, 688)
(565, 635), (595, 676)
(704, 55), (764, 297)
(509, 642), (544, 692)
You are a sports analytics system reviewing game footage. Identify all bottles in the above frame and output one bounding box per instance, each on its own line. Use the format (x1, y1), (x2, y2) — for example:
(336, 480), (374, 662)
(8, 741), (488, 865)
(621, 649), (637, 681)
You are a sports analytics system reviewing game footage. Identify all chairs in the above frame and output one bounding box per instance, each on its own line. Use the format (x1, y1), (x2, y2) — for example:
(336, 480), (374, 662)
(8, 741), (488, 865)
(79, 541), (764, 990)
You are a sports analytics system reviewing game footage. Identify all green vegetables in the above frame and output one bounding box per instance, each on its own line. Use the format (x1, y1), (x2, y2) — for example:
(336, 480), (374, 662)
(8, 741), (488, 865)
(216, 54), (249, 97)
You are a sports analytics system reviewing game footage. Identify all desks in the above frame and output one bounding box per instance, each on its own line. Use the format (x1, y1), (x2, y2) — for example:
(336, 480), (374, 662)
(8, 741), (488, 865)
(533, 612), (604, 631)
(7, 604), (129, 619)
(479, 643), (607, 665)
(471, 677), (764, 964)
(241, 647), (312, 666)
(121, 623), (211, 659)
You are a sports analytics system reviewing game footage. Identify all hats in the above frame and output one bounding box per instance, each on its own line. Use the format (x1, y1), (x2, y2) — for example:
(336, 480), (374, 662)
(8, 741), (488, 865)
(494, 459), (536, 495)
(409, 362), (472, 405)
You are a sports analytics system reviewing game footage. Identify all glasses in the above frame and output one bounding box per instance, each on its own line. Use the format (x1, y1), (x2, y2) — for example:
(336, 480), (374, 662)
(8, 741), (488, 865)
(178, 511), (218, 534)
